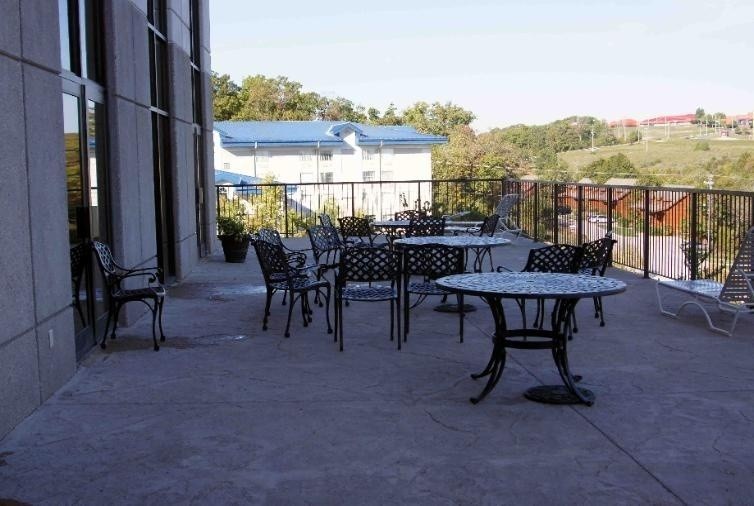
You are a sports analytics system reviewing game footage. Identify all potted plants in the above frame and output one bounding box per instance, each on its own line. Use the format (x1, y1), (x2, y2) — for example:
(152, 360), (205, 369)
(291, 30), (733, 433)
(213, 214), (253, 263)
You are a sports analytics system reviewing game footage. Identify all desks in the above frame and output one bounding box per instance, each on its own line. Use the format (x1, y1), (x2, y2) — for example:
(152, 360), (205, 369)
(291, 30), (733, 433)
(431, 270), (629, 407)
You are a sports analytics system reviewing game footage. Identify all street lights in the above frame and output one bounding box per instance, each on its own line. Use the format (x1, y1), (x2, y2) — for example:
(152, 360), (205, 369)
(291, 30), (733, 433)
(703, 174), (714, 253)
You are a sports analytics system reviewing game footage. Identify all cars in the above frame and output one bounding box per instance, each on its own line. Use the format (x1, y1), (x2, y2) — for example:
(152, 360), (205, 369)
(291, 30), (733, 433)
(557, 202), (571, 216)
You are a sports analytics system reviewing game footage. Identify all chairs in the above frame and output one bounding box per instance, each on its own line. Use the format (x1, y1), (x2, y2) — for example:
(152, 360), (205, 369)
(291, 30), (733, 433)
(655, 223), (753, 337)
(90, 239), (168, 353)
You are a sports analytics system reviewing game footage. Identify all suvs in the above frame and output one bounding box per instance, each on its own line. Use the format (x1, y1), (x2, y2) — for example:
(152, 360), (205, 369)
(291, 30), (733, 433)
(588, 213), (613, 224)
